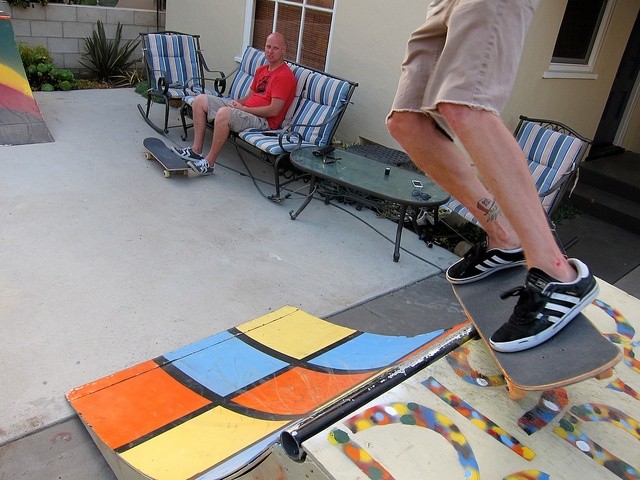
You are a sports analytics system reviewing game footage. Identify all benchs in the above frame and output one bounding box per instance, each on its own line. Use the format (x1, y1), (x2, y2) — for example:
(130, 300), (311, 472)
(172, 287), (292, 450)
(180, 46), (358, 199)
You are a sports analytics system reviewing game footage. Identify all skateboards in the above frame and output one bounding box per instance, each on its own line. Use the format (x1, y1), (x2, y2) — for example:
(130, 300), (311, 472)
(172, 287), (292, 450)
(453, 263), (621, 400)
(144, 137), (192, 178)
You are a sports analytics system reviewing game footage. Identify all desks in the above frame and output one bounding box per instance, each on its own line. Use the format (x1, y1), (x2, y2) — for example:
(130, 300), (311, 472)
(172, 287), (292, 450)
(289, 146), (451, 261)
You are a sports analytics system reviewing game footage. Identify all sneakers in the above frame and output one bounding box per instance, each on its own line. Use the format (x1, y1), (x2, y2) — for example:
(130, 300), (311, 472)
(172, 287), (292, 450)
(171, 146), (204, 162)
(187, 159), (215, 175)
(488, 257), (601, 353)
(445, 235), (527, 285)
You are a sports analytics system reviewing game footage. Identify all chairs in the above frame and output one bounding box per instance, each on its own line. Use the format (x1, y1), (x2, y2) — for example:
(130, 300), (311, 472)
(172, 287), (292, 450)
(136, 31), (224, 134)
(445, 115), (593, 242)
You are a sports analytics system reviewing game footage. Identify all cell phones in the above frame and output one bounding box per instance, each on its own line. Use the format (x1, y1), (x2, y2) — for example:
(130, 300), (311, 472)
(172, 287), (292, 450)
(261, 132), (278, 135)
(412, 179), (423, 188)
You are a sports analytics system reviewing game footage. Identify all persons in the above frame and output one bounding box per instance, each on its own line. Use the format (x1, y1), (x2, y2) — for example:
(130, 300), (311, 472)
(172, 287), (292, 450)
(171, 31), (296, 176)
(382, 0), (599, 354)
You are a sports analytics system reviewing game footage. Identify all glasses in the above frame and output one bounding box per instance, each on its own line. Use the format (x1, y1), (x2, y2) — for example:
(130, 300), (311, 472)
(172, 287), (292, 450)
(412, 190), (431, 200)
(321, 153), (342, 164)
